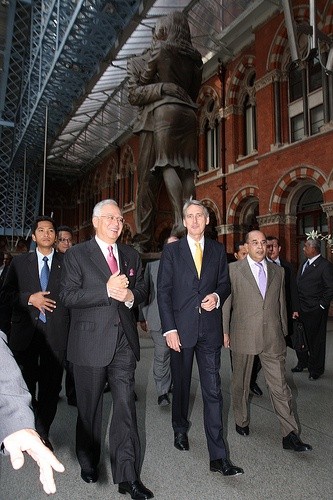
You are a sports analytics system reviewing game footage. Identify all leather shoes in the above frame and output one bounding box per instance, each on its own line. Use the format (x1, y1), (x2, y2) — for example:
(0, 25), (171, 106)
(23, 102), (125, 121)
(118, 479), (154, 500)
(81, 468), (98, 483)
(174, 432), (189, 451)
(210, 458), (244, 477)
(236, 424), (250, 436)
(282, 431), (312, 452)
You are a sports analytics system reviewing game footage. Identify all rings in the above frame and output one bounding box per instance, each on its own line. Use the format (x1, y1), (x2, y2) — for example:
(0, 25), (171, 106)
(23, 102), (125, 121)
(124, 281), (127, 285)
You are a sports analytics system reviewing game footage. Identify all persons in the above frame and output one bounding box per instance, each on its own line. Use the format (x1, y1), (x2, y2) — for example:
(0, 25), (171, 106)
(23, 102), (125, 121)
(58, 199), (155, 500)
(156, 200), (246, 478)
(103, 229), (180, 406)
(0, 329), (66, 495)
(126, 10), (204, 261)
(222, 230), (313, 453)
(0, 217), (74, 451)
(296, 238), (333, 380)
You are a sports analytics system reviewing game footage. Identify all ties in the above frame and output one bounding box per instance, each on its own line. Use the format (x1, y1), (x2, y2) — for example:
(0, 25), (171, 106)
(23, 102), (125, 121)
(107, 245), (118, 275)
(255, 262), (266, 298)
(38, 257), (51, 323)
(301, 261), (309, 278)
(193, 244), (203, 279)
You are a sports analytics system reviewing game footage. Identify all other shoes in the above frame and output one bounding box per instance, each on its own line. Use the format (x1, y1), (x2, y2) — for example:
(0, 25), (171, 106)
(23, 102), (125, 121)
(290, 361), (309, 373)
(250, 381), (264, 395)
(308, 371), (324, 381)
(158, 393), (170, 407)
(41, 436), (55, 450)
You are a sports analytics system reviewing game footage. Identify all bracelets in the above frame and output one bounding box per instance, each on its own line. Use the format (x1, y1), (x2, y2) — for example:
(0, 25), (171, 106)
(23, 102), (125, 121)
(128, 298), (134, 303)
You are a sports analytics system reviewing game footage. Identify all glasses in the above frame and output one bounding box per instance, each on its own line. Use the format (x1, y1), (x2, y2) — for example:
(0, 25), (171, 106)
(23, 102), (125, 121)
(58, 237), (74, 243)
(249, 241), (266, 246)
(99, 215), (126, 224)
